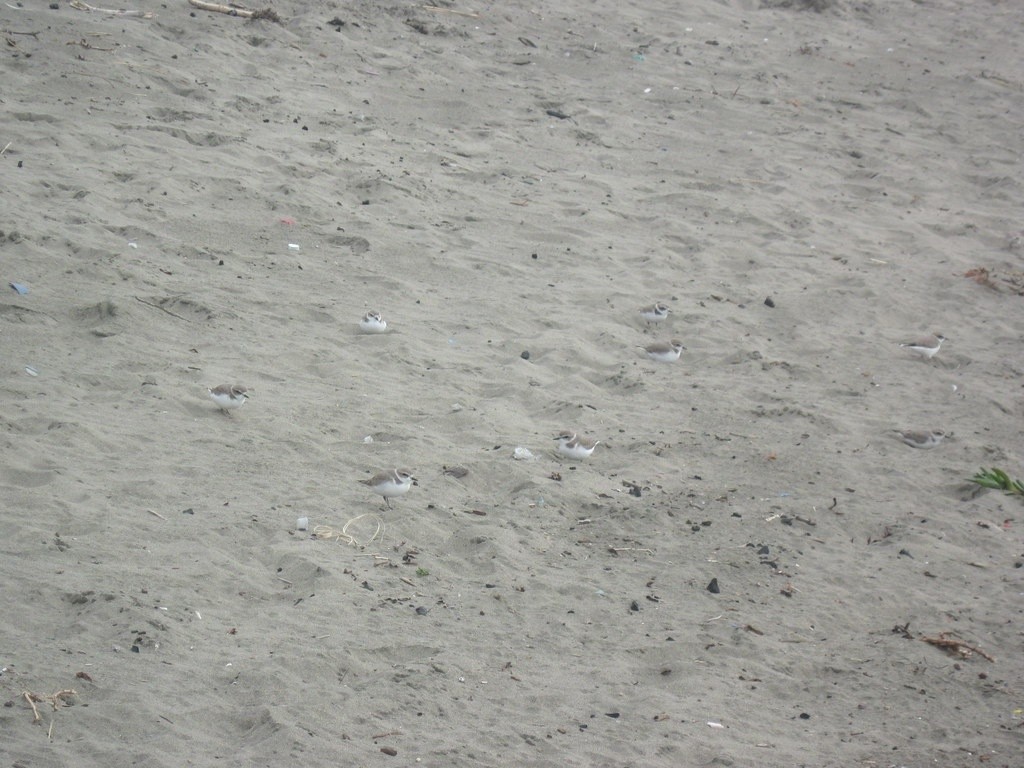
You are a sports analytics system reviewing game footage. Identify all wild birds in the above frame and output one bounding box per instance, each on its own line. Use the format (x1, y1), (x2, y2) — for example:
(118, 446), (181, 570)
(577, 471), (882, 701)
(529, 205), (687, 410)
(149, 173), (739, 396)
(356, 466), (419, 511)
(205, 383), (249, 418)
(358, 310), (393, 334)
(637, 303), (672, 327)
(635, 338), (687, 363)
(552, 430), (602, 462)
(887, 331), (951, 367)
(888, 428), (951, 450)
